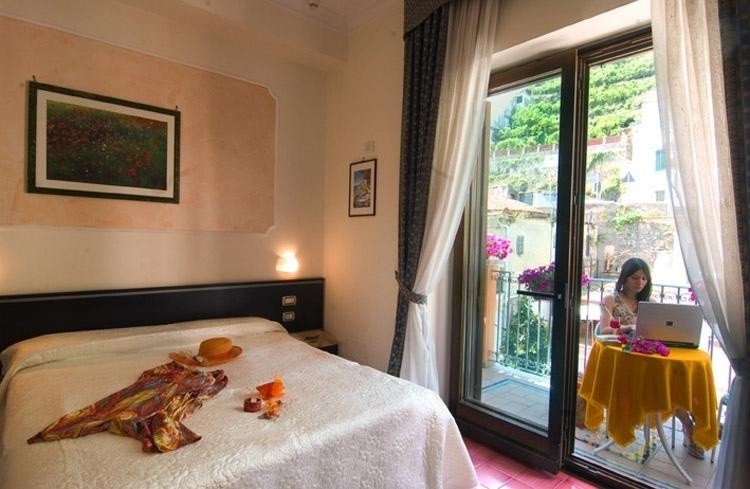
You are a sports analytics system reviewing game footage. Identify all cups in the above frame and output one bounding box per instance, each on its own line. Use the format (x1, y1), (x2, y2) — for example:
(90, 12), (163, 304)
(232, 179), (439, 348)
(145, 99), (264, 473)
(609, 316), (621, 339)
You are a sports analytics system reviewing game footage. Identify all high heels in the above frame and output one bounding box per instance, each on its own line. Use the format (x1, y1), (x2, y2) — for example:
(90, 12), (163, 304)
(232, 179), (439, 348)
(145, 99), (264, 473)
(682, 427), (705, 459)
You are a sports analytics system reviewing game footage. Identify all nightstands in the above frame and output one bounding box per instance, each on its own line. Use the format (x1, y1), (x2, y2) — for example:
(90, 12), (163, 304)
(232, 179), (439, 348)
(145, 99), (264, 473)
(290, 332), (342, 355)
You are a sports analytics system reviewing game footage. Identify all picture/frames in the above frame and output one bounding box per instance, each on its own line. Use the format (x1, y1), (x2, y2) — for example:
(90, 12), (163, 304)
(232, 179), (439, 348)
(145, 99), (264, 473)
(27, 82), (186, 207)
(348, 159), (377, 218)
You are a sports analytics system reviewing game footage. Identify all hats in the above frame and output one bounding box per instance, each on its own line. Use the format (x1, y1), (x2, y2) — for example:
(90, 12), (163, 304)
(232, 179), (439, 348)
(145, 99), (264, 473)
(192, 338), (243, 366)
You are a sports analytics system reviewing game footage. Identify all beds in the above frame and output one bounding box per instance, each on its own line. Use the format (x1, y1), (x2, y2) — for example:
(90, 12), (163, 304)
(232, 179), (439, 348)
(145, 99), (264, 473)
(0, 277), (482, 489)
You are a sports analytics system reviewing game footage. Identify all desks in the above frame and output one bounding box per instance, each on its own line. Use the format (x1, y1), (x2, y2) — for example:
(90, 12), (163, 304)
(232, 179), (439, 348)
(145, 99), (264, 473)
(592, 336), (709, 486)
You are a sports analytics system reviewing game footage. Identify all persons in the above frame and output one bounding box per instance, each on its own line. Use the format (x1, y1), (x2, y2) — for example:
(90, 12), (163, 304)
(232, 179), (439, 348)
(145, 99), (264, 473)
(597, 259), (726, 460)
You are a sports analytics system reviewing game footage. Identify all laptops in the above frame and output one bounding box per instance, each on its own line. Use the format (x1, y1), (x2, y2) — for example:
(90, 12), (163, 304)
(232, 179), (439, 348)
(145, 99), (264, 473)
(635, 301), (703, 350)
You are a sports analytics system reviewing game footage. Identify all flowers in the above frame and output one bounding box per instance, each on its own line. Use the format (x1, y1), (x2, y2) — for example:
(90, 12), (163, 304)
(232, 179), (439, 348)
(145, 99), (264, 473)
(517, 261), (591, 293)
(618, 333), (670, 358)
(486, 233), (512, 261)
